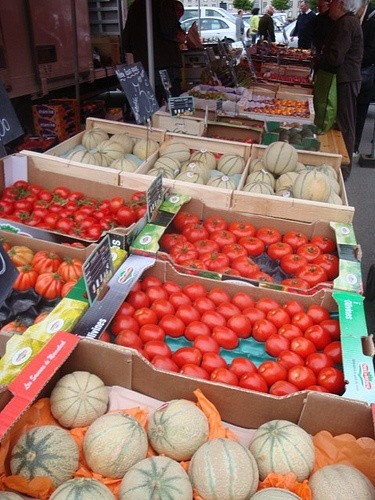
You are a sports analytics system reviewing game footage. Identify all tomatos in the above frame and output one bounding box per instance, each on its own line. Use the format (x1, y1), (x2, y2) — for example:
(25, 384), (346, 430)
(1, 320), (27, 336)
(34, 311), (50, 324)
(160, 211), (339, 289)
(100, 277), (345, 396)
(0, 237), (84, 299)
(0, 180), (147, 241)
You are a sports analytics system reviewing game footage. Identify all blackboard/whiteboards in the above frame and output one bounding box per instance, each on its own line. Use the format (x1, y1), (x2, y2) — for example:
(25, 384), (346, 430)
(116, 61), (161, 123)
(0, 81), (24, 144)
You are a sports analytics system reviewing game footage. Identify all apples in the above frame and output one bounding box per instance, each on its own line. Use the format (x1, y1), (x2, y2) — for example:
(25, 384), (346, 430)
(210, 122), (262, 144)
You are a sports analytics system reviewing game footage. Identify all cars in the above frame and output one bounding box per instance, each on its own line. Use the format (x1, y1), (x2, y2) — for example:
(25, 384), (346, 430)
(283, 19), (298, 48)
(179, 16), (252, 50)
(238, 13), (288, 46)
(177, 7), (250, 28)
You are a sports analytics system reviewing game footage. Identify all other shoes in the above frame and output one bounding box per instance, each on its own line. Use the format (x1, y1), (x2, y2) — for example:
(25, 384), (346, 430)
(352, 150), (359, 157)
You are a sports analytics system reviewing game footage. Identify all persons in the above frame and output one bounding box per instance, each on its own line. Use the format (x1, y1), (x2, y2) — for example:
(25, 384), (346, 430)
(352, 0), (375, 158)
(124, 0), (185, 108)
(291, 0), (316, 49)
(258, 5), (275, 42)
(249, 8), (260, 44)
(311, 0), (363, 181)
(236, 10), (245, 40)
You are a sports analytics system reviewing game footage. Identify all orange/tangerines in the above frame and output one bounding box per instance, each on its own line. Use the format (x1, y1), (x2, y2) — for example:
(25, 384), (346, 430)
(244, 98), (309, 116)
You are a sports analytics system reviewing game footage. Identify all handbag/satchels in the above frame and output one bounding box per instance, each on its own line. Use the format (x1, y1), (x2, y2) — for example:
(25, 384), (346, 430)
(313, 67), (339, 136)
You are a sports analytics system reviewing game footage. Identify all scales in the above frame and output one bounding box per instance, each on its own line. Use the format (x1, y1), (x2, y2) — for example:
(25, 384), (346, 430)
(183, 49), (208, 66)
